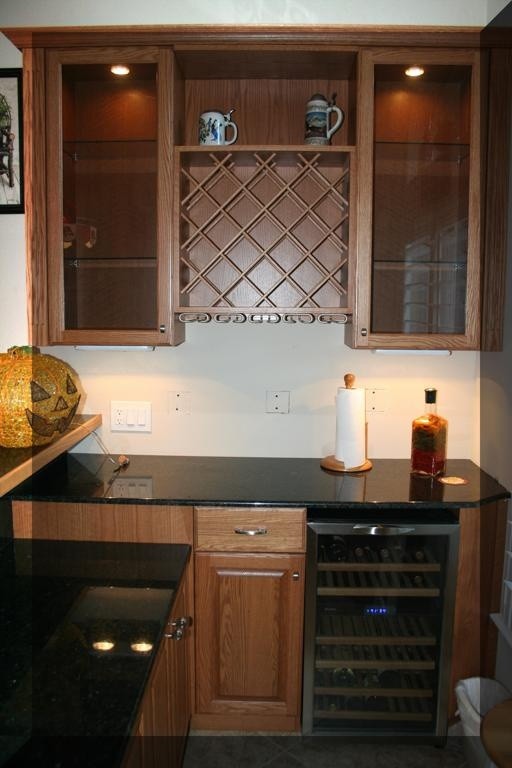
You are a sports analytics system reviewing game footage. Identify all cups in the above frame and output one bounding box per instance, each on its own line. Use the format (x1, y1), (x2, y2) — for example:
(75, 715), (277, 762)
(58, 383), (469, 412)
(304, 94), (343, 145)
(197, 110), (237, 147)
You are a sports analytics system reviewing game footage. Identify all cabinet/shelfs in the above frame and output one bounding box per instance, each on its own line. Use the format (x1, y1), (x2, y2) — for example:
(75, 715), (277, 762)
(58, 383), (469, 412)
(121, 556), (194, 768)
(191, 505), (307, 734)
(0, 22), (484, 353)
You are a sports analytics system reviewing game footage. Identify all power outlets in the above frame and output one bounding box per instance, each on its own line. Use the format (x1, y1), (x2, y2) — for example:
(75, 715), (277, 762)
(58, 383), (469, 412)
(109, 400), (153, 434)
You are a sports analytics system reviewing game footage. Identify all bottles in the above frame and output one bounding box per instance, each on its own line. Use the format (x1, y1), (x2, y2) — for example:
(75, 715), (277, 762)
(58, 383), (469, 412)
(410, 386), (449, 478)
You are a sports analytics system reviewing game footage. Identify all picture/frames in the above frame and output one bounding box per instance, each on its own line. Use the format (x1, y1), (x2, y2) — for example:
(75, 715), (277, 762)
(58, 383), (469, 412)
(0, 66), (27, 214)
